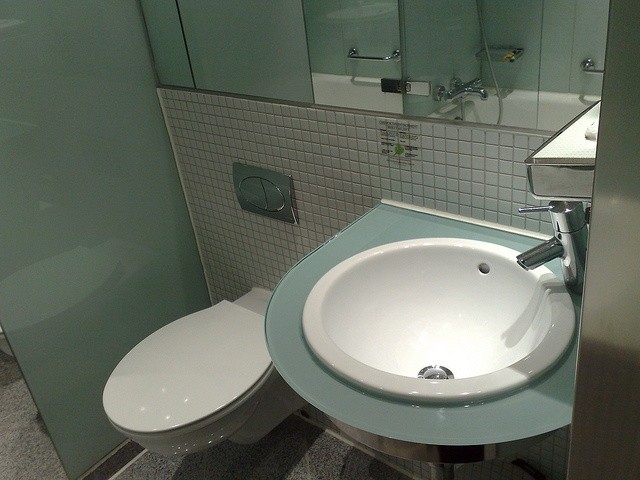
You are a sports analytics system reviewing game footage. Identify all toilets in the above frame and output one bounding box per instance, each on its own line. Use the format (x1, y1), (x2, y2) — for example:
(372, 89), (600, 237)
(103, 288), (309, 456)
(0, 238), (135, 358)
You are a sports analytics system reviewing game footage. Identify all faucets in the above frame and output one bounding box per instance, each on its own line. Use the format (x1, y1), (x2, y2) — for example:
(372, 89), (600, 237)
(515, 201), (588, 295)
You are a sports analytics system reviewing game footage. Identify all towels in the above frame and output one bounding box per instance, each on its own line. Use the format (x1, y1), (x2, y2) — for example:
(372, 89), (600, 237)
(311, 70), (403, 116)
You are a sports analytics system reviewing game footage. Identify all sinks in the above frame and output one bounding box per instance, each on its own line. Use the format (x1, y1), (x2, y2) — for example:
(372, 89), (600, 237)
(302, 237), (577, 404)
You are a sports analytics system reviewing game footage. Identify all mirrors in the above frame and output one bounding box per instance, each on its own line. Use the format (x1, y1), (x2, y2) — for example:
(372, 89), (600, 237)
(399, 0), (611, 134)
(176, 0), (314, 107)
(139, 0), (196, 91)
(300, 0), (405, 117)
